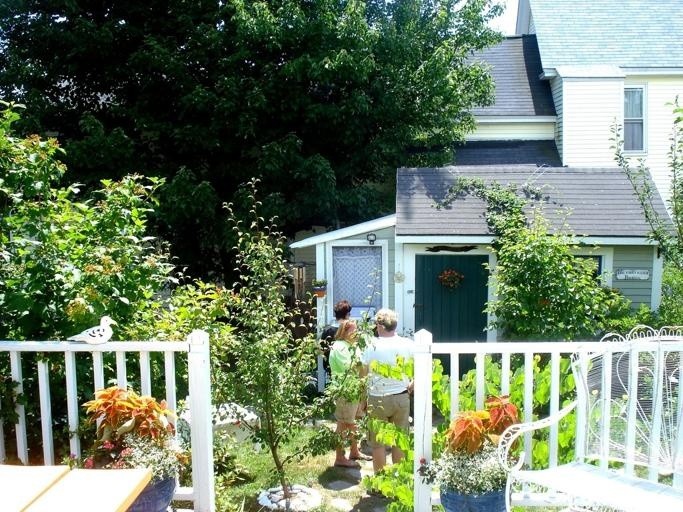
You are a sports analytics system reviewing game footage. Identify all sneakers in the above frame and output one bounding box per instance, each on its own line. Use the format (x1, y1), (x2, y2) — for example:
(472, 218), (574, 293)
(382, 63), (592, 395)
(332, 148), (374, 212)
(333, 451), (372, 468)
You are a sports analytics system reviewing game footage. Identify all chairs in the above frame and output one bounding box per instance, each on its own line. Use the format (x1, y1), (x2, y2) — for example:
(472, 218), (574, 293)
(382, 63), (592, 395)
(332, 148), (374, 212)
(498, 323), (683, 511)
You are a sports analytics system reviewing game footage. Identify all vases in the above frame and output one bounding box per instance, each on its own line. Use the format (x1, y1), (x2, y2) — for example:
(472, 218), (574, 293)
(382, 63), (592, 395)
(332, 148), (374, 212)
(439, 478), (507, 511)
(93, 454), (174, 512)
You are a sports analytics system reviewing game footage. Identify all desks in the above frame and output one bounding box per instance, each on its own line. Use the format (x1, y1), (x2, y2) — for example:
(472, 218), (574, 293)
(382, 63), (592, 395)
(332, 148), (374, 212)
(180, 402), (262, 454)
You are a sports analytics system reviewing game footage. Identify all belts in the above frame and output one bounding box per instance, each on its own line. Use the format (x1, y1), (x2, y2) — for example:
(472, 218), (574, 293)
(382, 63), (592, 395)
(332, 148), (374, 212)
(392, 389), (408, 395)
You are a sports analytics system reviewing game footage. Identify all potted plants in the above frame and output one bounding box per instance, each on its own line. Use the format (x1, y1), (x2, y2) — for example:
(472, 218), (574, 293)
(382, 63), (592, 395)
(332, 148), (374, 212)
(311, 278), (327, 297)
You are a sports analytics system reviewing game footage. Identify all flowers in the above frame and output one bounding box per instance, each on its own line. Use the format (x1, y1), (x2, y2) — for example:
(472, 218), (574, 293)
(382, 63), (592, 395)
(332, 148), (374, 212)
(437, 267), (465, 293)
(415, 392), (531, 498)
(59, 386), (192, 487)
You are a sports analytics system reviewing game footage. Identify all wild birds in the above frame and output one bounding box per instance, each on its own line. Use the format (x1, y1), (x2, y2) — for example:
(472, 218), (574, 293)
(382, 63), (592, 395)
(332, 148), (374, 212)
(66, 316), (118, 344)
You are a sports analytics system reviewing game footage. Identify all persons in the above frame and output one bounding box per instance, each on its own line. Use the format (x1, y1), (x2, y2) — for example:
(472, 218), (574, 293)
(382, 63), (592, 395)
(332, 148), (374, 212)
(320, 298), (352, 375)
(359, 307), (414, 476)
(329, 320), (373, 469)
